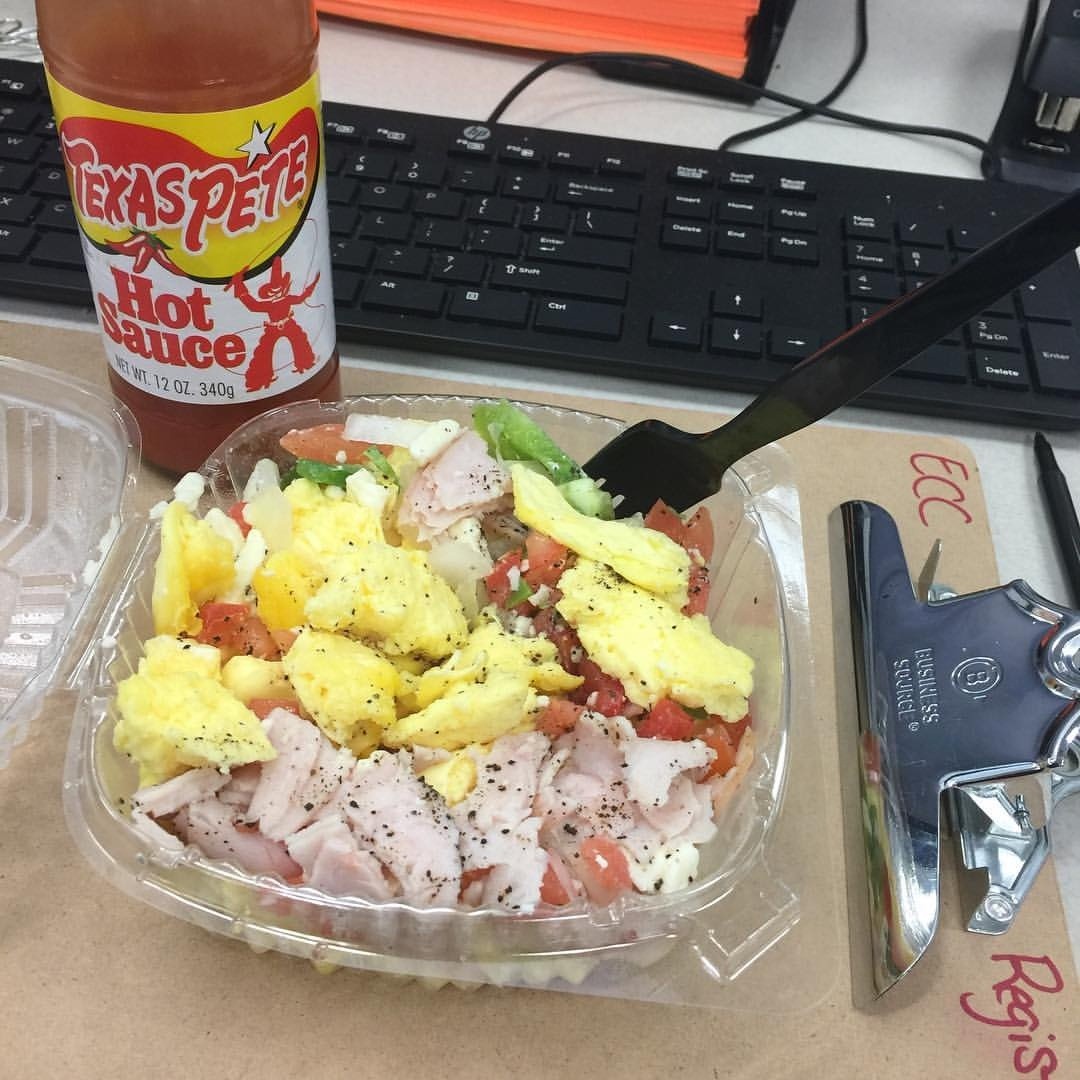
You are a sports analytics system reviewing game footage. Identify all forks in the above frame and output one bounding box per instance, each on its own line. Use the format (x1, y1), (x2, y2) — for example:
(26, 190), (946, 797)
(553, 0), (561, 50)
(575, 181), (1077, 517)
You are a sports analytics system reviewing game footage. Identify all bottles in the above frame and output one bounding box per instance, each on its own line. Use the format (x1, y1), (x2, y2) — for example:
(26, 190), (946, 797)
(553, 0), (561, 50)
(34, 1), (368, 460)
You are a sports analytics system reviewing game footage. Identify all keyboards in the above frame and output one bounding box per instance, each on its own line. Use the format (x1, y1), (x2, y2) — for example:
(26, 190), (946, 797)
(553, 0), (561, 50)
(2, 57), (1080, 429)
